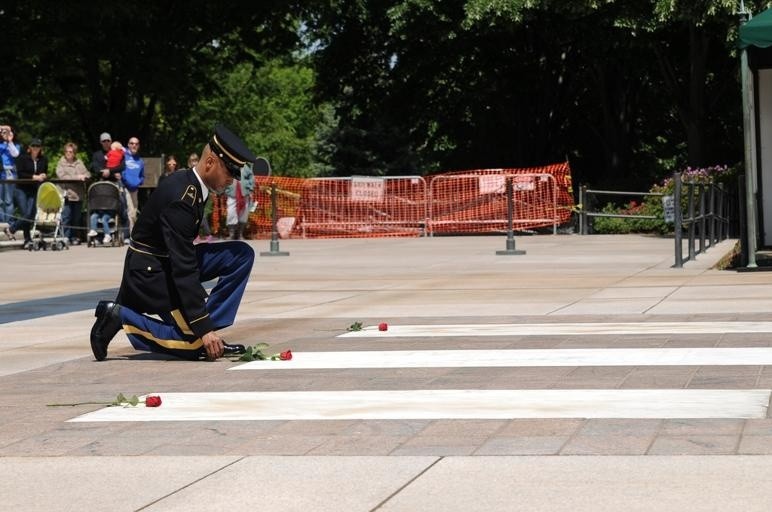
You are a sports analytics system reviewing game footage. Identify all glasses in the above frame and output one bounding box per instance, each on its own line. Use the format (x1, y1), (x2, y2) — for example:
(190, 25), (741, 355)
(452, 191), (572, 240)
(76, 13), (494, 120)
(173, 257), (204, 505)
(31, 144), (40, 147)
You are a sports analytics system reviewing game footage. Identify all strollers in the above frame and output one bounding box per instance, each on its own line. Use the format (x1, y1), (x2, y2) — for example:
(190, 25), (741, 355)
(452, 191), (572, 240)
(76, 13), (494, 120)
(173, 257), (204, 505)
(21, 181), (71, 252)
(84, 175), (125, 249)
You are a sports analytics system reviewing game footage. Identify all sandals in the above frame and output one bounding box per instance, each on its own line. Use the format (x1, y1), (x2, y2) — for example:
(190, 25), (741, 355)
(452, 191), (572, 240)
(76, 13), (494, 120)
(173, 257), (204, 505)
(4, 227), (16, 241)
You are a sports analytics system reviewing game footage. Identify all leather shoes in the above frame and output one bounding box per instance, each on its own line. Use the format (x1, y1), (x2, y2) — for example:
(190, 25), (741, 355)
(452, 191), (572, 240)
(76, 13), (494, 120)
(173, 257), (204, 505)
(90, 301), (117, 361)
(202, 340), (246, 355)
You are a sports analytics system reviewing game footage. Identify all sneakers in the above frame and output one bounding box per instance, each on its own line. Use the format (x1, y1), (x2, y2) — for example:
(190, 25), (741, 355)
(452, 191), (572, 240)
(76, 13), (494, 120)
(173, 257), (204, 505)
(87, 230), (98, 236)
(193, 235), (201, 244)
(103, 234), (112, 243)
(204, 235), (213, 242)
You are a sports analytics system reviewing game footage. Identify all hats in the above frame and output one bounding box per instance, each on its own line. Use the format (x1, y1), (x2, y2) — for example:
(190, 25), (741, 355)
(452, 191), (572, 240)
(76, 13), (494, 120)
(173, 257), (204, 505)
(30, 138), (42, 146)
(209, 124), (256, 181)
(100, 132), (112, 142)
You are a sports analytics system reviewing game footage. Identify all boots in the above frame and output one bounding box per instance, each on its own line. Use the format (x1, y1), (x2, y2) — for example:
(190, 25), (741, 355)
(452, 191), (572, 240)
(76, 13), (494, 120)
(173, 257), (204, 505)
(238, 223), (246, 241)
(225, 225), (236, 240)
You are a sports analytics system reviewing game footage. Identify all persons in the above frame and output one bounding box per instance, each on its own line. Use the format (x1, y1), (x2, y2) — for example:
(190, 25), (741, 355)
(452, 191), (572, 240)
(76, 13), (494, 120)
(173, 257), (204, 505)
(90, 132), (131, 244)
(121, 137), (145, 239)
(87, 209), (115, 243)
(0, 124), (22, 222)
(218, 163), (256, 241)
(4, 137), (48, 248)
(187, 152), (212, 244)
(99, 141), (126, 192)
(158, 154), (181, 184)
(90, 124), (258, 361)
(55, 142), (91, 245)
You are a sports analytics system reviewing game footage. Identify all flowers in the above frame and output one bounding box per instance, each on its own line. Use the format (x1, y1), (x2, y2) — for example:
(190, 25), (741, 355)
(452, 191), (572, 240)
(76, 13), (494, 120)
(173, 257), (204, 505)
(313, 322), (388, 331)
(46, 393), (161, 407)
(221, 343), (292, 361)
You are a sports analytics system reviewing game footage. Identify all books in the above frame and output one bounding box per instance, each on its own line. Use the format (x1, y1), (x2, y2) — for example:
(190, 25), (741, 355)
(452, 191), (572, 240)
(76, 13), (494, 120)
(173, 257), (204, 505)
(248, 201), (258, 212)
